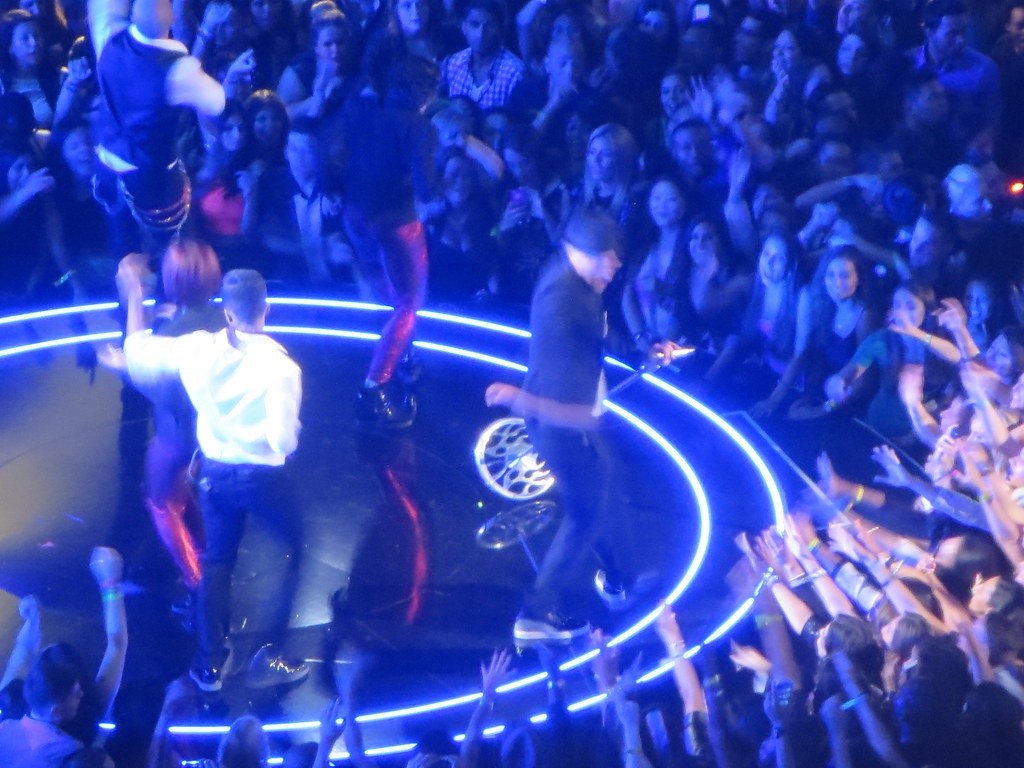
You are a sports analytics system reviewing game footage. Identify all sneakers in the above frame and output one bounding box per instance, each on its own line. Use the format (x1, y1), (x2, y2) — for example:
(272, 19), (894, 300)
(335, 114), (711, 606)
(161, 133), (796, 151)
(595, 569), (625, 603)
(514, 612), (590, 639)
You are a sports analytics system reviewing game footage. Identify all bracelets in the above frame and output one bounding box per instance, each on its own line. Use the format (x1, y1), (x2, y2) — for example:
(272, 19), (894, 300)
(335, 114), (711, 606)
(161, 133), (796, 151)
(101, 580), (123, 601)
(546, 677), (565, 689)
(878, 574), (894, 588)
(787, 571), (806, 582)
(666, 640), (686, 655)
(754, 614), (783, 629)
(807, 538), (822, 554)
(476, 699), (495, 711)
(977, 491), (994, 505)
(881, 554), (895, 569)
(805, 568), (828, 581)
(956, 337), (974, 350)
(855, 481), (864, 507)
(924, 329), (934, 347)
(766, 575), (782, 588)
(892, 560), (904, 575)
(975, 461), (995, 476)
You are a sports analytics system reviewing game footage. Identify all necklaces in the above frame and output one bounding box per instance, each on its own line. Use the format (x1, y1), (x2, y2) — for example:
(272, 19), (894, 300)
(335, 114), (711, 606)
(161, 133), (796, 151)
(837, 306), (861, 329)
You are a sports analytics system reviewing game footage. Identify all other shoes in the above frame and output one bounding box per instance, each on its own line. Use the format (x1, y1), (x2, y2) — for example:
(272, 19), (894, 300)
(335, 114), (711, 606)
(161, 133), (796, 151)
(353, 384), (413, 429)
(190, 664), (223, 692)
(394, 344), (422, 382)
(244, 653), (309, 688)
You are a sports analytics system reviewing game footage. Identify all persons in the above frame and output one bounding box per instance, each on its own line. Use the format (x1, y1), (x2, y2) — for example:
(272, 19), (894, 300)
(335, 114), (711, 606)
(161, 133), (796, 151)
(0, 0), (1024, 768)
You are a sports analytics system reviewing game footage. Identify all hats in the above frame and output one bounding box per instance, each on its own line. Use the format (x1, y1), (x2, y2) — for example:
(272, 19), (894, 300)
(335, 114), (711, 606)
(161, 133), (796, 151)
(564, 211), (617, 251)
